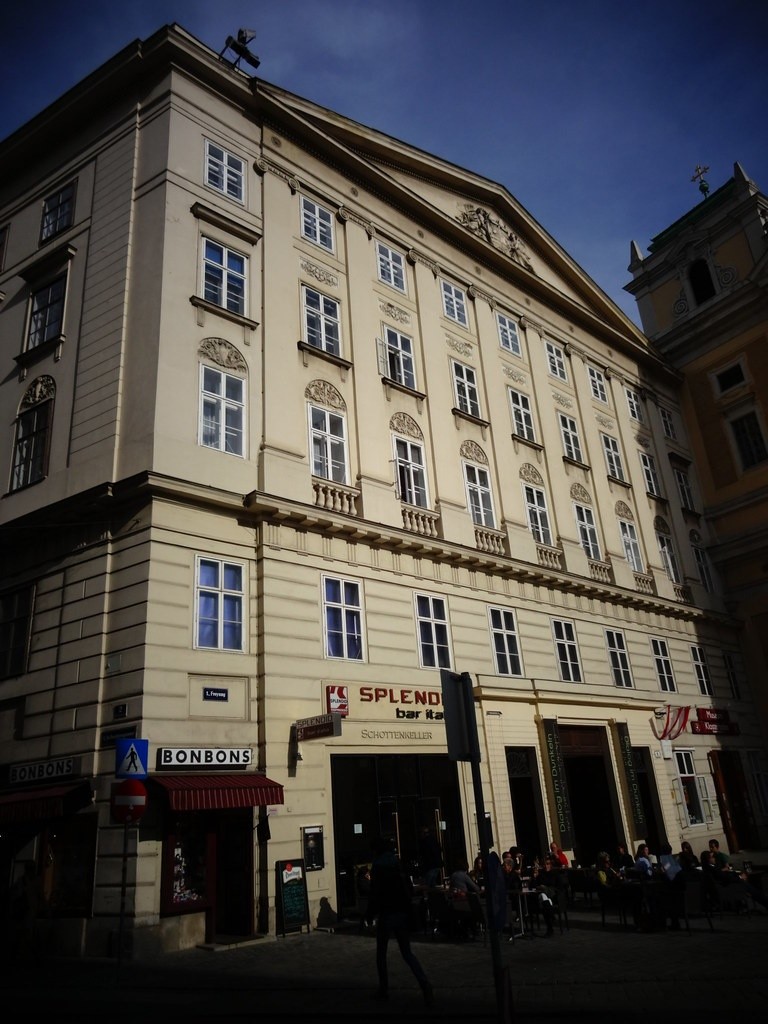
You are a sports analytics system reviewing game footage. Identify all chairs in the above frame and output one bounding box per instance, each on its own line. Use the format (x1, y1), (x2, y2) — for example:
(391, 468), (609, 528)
(411, 862), (768, 939)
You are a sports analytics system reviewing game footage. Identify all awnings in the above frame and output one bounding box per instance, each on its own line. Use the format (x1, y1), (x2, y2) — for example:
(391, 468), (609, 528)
(0, 785), (93, 822)
(148, 774), (283, 812)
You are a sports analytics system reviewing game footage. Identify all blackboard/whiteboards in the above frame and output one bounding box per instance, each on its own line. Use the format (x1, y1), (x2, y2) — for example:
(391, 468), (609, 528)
(277, 859), (311, 929)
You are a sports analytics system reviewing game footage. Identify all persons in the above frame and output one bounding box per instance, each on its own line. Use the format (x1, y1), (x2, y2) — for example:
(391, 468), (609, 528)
(358, 829), (436, 1006)
(14, 859), (41, 960)
(361, 840), (768, 941)
